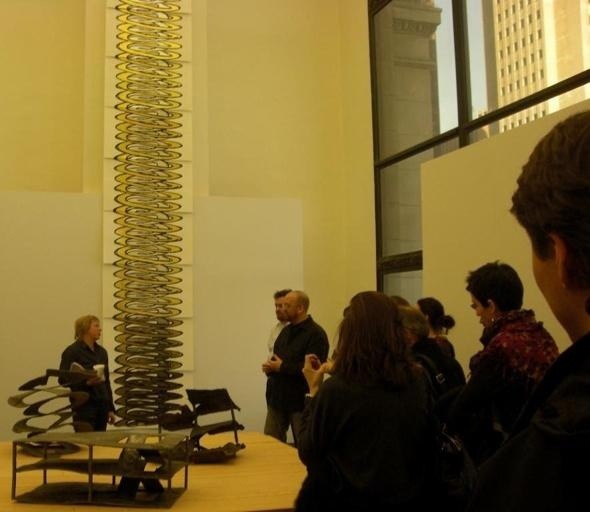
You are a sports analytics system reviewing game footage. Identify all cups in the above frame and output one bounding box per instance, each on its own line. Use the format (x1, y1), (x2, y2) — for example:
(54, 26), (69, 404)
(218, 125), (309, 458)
(93, 364), (105, 382)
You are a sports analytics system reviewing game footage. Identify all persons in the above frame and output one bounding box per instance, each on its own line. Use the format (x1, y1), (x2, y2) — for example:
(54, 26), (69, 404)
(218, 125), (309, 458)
(263, 262), (560, 510)
(57, 315), (116, 432)
(468, 111), (588, 510)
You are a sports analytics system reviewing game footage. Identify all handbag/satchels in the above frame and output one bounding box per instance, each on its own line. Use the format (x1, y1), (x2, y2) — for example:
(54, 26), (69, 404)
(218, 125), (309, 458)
(431, 421), (487, 512)
